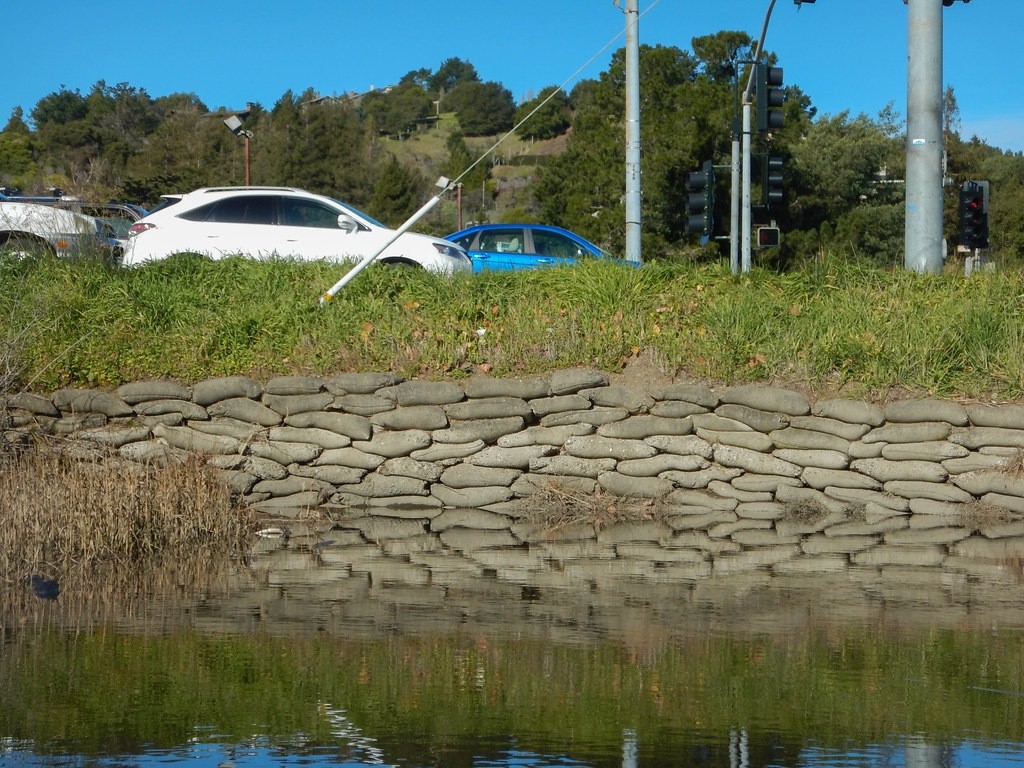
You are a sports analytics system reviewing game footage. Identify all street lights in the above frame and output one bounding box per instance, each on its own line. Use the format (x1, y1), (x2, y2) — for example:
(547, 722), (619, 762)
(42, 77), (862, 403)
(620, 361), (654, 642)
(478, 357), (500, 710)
(223, 114), (253, 189)
(435, 177), (461, 233)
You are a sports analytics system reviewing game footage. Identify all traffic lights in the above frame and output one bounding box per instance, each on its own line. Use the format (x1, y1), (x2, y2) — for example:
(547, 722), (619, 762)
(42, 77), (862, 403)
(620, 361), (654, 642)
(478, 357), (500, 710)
(757, 226), (781, 251)
(960, 180), (989, 249)
(755, 63), (785, 132)
(766, 155), (784, 205)
(684, 170), (713, 237)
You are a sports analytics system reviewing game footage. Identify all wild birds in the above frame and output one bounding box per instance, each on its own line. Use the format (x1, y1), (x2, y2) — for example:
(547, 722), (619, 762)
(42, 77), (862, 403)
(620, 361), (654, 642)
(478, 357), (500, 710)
(32, 574), (61, 596)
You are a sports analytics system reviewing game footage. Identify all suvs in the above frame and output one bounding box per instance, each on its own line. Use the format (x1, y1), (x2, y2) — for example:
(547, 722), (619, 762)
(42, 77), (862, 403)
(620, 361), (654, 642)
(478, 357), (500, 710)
(0, 184), (149, 237)
(121, 183), (473, 274)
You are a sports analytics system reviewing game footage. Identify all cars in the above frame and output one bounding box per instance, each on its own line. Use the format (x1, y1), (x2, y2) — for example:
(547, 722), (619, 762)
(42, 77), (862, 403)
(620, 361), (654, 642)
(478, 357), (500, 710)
(439, 221), (647, 277)
(0, 202), (121, 265)
